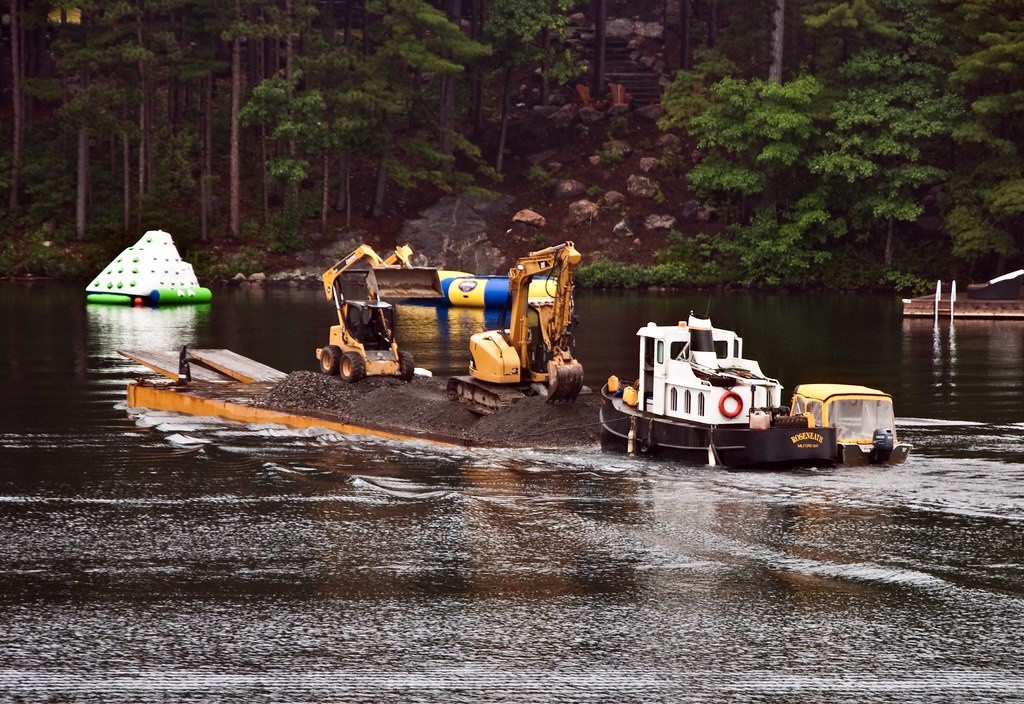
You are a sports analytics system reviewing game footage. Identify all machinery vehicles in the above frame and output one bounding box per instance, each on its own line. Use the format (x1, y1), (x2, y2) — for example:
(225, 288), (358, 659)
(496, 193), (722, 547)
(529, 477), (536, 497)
(315, 245), (448, 385)
(446, 241), (585, 418)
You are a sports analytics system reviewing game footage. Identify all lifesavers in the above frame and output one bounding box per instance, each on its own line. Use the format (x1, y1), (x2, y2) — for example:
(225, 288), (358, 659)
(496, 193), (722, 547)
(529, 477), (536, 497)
(718, 391), (744, 419)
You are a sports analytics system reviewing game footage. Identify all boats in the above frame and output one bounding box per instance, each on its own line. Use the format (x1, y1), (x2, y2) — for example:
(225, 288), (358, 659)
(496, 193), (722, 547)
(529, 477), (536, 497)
(599, 311), (838, 473)
(780, 382), (913, 468)
